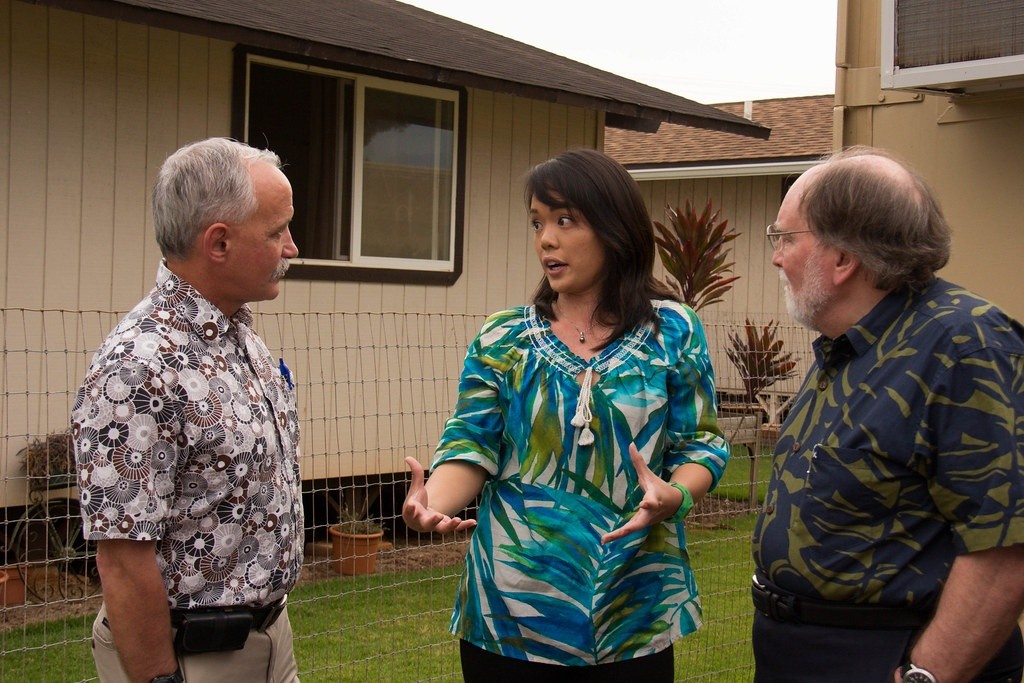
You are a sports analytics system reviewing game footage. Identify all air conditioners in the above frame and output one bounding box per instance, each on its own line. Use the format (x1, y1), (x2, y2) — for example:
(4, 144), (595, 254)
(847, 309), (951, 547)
(881, 0), (1024, 100)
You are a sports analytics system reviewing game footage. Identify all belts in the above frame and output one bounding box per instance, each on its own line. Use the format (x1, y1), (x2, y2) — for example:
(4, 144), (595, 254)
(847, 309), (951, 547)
(172, 593), (289, 634)
(750, 574), (929, 631)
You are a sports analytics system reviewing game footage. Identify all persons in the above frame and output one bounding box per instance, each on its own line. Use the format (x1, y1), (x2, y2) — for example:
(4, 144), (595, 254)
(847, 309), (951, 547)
(67, 135), (306, 683)
(401, 149), (730, 683)
(750, 145), (1024, 683)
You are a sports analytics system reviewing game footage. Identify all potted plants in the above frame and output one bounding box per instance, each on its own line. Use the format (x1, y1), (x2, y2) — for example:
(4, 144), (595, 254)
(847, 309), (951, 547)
(719, 318), (799, 413)
(330, 503), (383, 576)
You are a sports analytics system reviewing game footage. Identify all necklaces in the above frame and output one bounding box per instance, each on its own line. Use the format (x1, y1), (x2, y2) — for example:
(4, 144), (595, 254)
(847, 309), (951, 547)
(555, 300), (602, 344)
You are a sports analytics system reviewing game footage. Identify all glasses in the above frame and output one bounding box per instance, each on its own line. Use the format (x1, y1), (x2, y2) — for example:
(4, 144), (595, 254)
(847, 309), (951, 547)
(765, 225), (814, 252)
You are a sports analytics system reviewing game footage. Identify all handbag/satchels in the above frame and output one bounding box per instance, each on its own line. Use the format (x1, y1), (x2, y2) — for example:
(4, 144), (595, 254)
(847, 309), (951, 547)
(173, 614), (254, 654)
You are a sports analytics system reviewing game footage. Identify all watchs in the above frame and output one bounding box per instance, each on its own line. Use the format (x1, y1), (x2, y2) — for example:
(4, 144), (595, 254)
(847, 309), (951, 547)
(148, 665), (184, 683)
(900, 658), (937, 683)
(663, 481), (694, 525)
